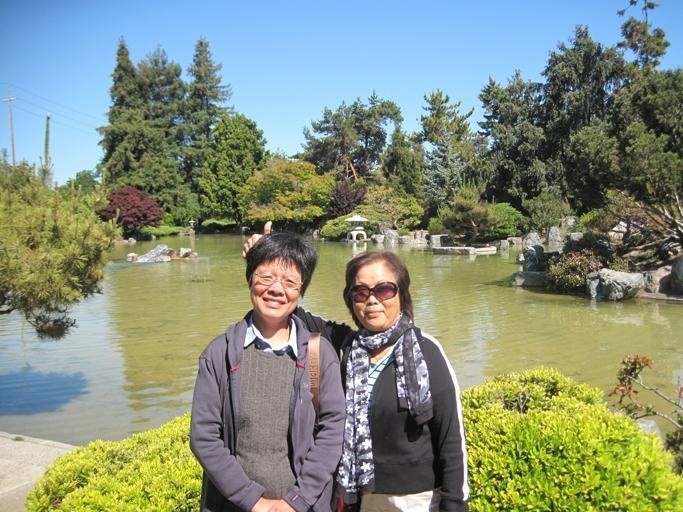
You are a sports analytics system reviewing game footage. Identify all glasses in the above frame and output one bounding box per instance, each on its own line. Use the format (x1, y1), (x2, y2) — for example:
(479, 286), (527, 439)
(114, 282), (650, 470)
(252, 273), (304, 291)
(349, 281), (399, 303)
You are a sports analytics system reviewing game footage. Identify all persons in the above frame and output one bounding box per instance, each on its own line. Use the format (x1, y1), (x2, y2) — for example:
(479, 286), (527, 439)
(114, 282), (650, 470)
(187, 230), (347, 512)
(239, 220), (469, 511)
